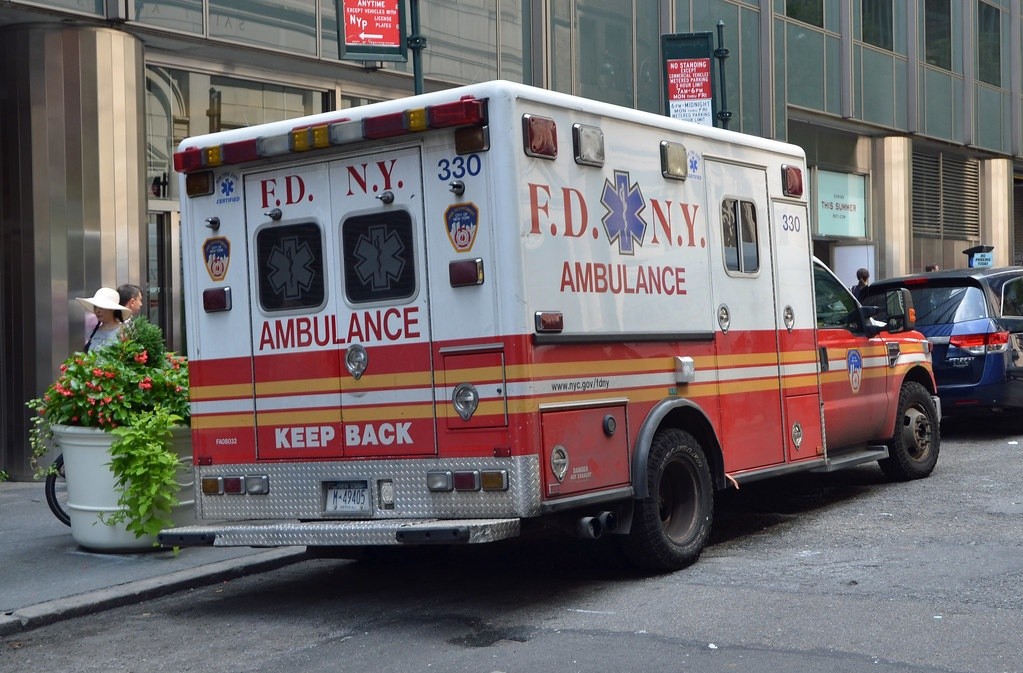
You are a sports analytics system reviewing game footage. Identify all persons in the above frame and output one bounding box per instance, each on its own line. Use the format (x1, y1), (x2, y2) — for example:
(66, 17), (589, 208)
(848, 268), (871, 299)
(116, 283), (144, 324)
(925, 262), (940, 273)
(75, 287), (133, 357)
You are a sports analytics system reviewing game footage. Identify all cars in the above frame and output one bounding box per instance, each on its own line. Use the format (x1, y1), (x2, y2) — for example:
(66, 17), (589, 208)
(856, 267), (1023, 429)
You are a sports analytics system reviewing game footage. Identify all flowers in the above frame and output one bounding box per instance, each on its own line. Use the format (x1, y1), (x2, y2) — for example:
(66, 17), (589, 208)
(26, 315), (191, 559)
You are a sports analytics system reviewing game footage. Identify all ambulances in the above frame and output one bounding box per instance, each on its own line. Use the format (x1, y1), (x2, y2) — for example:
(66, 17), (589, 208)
(154, 76), (944, 576)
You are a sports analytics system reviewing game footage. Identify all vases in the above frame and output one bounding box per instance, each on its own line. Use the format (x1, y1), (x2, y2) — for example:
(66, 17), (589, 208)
(46, 422), (198, 552)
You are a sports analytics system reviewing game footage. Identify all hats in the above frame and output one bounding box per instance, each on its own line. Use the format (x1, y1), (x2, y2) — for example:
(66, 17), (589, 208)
(76, 287), (133, 322)
